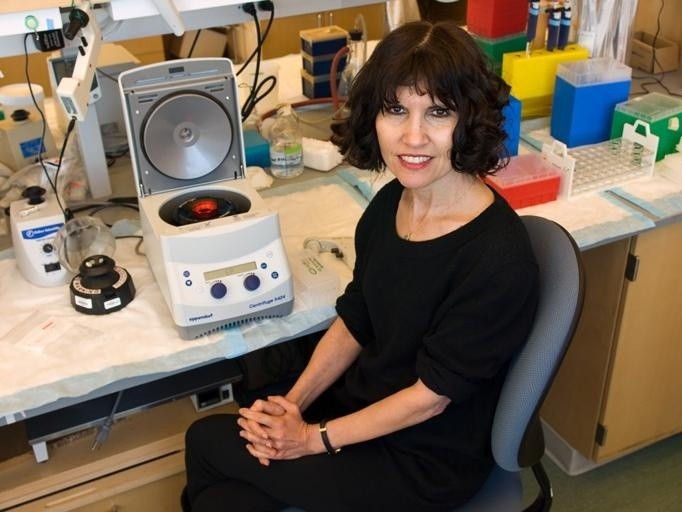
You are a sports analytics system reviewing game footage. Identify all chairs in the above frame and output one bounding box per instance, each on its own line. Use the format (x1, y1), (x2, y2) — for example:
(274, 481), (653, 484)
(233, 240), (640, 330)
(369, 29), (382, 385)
(456, 216), (586, 512)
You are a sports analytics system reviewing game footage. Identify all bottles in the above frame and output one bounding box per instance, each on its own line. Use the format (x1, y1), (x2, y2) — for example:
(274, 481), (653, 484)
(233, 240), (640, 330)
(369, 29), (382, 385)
(524, 2), (571, 57)
(336, 28), (364, 96)
(268, 103), (305, 179)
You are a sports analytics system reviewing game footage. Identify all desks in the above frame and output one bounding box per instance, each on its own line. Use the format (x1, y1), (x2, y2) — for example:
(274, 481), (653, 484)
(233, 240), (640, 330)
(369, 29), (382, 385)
(0, 38), (682, 512)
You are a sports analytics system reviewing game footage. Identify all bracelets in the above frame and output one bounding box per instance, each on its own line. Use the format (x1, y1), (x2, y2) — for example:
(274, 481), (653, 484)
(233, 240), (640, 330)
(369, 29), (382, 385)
(318, 418), (340, 457)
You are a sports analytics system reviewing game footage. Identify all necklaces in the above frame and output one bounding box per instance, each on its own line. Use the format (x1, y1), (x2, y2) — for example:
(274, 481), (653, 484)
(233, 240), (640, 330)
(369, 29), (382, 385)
(404, 179), (476, 241)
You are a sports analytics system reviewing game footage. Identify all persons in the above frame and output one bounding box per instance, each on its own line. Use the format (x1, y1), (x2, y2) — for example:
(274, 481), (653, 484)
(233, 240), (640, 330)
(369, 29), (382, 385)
(180, 18), (538, 511)
